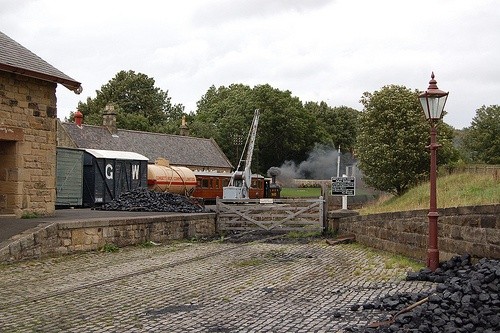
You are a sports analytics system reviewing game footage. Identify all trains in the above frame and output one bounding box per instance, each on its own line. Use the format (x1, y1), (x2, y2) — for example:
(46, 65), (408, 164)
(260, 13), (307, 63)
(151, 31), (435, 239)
(52, 146), (282, 208)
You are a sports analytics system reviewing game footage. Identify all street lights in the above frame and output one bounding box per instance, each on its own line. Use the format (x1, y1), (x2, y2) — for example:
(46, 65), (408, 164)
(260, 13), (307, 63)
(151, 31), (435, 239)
(415, 72), (451, 274)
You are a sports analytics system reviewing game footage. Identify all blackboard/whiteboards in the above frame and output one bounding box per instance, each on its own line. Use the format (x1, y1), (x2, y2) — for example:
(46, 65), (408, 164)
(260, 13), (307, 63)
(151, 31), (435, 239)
(330, 176), (356, 197)
